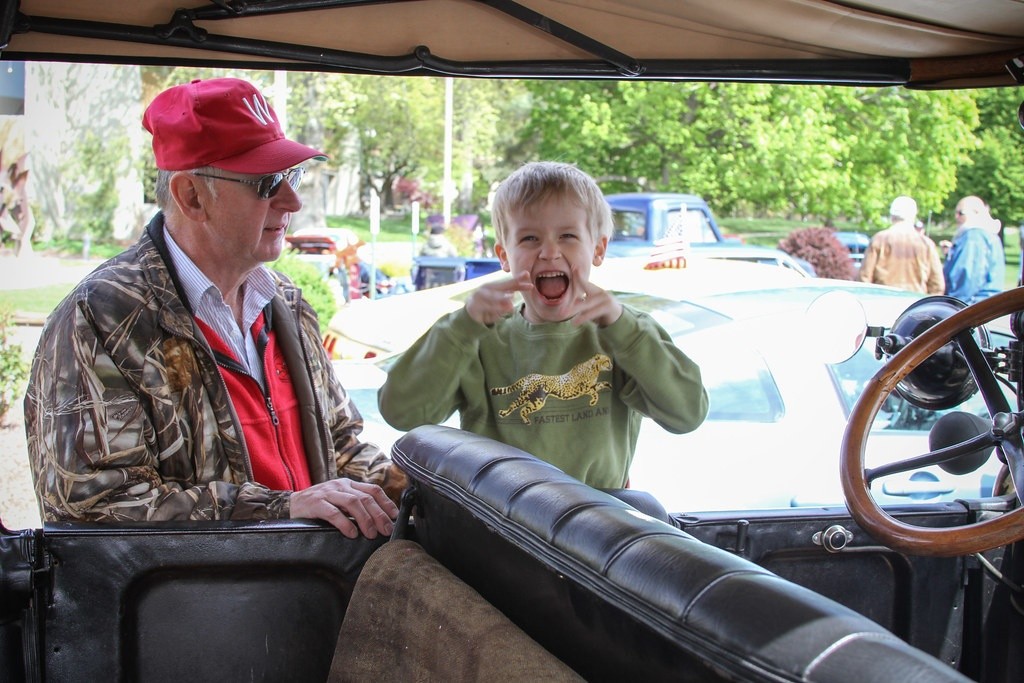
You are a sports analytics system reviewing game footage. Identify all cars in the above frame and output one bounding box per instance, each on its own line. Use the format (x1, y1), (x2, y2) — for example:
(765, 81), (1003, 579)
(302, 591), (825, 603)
(323, 247), (1018, 512)
(286, 226), (361, 254)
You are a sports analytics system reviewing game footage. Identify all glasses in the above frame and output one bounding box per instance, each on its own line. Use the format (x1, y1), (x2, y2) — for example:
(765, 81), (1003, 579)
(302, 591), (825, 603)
(193, 166), (305, 200)
(956, 210), (965, 215)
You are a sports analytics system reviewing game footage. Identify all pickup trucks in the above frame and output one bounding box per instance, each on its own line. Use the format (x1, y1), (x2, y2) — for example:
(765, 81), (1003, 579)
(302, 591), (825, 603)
(409, 194), (744, 290)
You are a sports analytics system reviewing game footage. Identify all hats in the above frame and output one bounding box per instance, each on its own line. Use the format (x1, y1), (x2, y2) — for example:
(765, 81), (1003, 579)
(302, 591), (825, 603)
(142, 78), (329, 174)
(890, 196), (917, 224)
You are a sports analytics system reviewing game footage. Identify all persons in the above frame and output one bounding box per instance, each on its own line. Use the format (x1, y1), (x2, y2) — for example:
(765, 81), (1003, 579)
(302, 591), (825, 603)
(376, 162), (709, 487)
(860, 196), (946, 296)
(24, 78), (408, 538)
(939, 196), (1005, 303)
(419, 226), (465, 282)
(780, 228), (854, 280)
